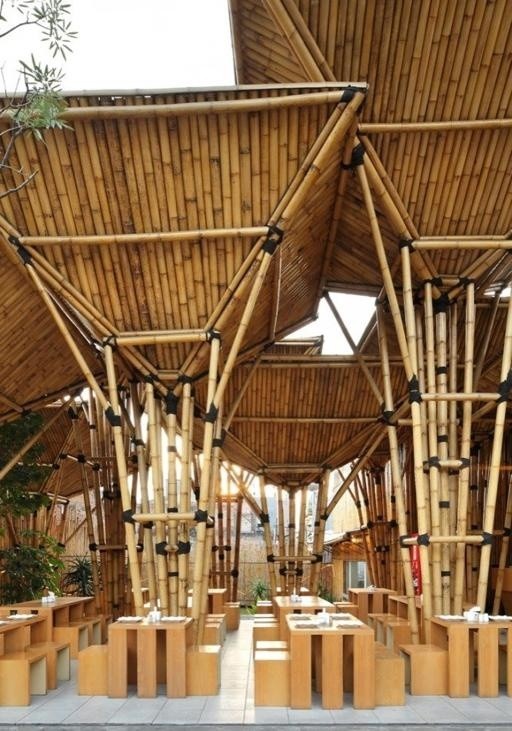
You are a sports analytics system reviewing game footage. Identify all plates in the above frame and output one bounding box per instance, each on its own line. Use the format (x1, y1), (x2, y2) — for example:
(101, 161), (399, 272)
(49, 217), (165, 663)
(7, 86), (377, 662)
(284, 609), (365, 629)
(6, 613), (38, 620)
(434, 606), (511, 624)
(161, 615), (188, 622)
(116, 615), (143, 624)
(0, 620), (9, 625)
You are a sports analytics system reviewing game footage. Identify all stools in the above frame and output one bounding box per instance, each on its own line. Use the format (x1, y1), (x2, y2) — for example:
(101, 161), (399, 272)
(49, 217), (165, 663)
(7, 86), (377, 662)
(253, 588), (511, 711)
(0, 588), (240, 707)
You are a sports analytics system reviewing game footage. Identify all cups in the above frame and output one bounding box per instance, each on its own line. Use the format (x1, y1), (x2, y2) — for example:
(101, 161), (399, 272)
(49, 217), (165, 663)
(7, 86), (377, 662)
(364, 582), (376, 592)
(42, 590), (80, 604)
(289, 593), (303, 603)
(146, 606), (162, 625)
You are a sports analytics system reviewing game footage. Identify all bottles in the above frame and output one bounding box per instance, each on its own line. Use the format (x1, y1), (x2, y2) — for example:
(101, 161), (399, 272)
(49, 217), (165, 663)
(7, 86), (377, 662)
(420, 593), (424, 604)
